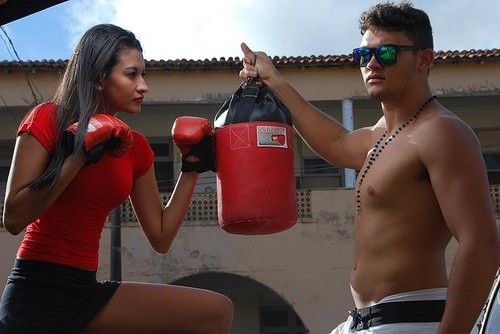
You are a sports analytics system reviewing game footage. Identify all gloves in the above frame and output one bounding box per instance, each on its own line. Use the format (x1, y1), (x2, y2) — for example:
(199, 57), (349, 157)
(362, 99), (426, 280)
(170, 113), (216, 175)
(62, 110), (133, 166)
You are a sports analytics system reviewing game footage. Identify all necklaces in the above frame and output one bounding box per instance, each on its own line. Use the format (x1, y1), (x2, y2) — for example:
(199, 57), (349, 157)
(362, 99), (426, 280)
(356, 95), (436, 217)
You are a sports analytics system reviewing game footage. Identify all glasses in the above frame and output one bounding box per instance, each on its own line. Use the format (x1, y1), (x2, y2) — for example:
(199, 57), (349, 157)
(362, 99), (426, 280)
(352, 42), (418, 68)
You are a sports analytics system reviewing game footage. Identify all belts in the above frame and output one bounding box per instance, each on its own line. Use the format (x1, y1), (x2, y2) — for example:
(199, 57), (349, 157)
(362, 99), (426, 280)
(344, 298), (449, 330)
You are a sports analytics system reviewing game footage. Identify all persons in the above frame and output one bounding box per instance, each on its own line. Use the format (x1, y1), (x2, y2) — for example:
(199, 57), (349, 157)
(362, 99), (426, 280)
(0, 23), (235, 334)
(240, 0), (499, 334)
